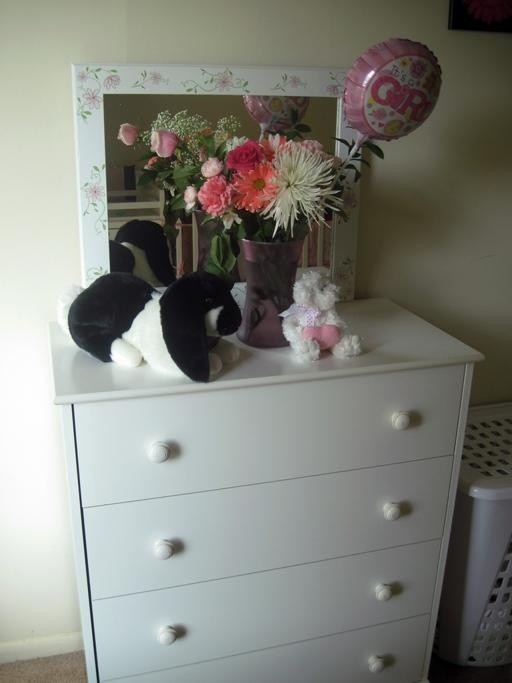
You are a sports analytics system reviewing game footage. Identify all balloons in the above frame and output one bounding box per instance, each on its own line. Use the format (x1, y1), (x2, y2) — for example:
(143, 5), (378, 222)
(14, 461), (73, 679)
(340, 35), (444, 142)
(242, 94), (310, 132)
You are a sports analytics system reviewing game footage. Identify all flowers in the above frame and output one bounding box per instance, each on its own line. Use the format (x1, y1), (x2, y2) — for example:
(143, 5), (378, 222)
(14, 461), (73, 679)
(198, 131), (385, 244)
(113, 109), (312, 274)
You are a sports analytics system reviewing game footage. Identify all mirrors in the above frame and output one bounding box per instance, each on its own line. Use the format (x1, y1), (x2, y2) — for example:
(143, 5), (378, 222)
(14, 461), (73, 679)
(77, 61), (366, 298)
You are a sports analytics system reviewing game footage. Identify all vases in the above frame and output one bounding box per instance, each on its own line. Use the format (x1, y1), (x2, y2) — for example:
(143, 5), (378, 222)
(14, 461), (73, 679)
(171, 220), (208, 286)
(236, 234), (293, 349)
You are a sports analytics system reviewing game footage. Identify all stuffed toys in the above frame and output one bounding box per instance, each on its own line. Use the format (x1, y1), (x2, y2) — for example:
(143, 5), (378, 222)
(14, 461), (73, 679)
(278, 270), (363, 364)
(65, 268), (243, 384)
(108, 218), (179, 286)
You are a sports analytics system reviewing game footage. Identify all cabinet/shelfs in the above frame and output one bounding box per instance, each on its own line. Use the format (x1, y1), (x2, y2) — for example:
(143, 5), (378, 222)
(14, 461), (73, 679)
(46, 299), (484, 683)
